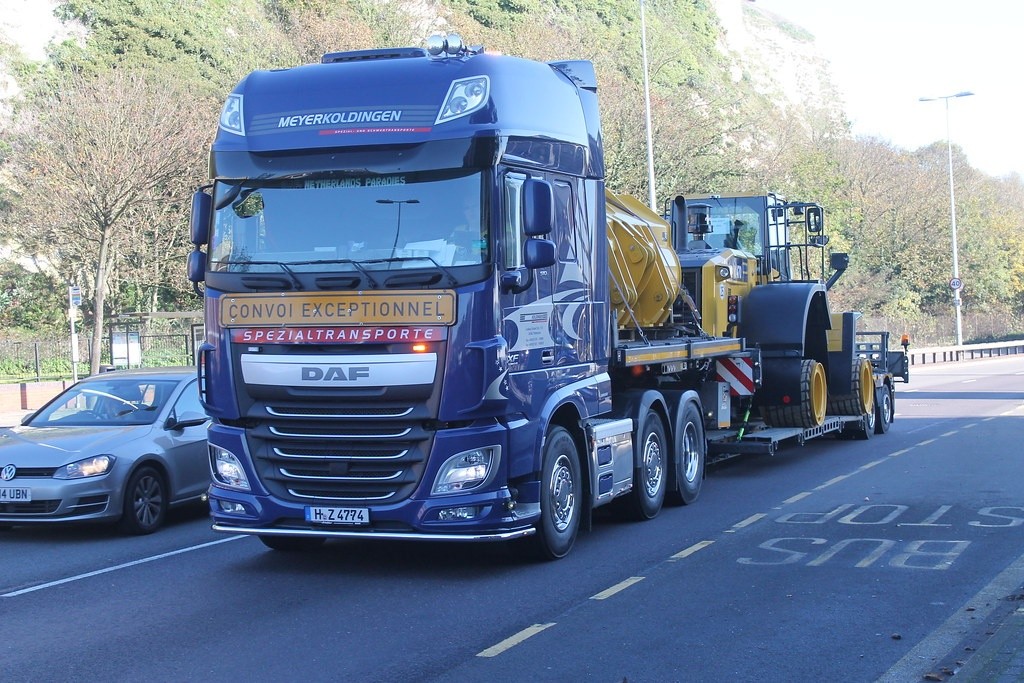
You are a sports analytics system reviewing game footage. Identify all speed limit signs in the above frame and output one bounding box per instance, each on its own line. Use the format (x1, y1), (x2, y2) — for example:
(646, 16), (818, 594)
(950, 278), (962, 289)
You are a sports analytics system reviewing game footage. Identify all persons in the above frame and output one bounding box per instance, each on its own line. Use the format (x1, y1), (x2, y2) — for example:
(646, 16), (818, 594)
(446, 196), (479, 239)
(94, 391), (123, 419)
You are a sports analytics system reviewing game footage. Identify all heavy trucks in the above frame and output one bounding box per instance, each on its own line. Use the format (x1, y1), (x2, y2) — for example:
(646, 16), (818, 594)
(184, 36), (911, 560)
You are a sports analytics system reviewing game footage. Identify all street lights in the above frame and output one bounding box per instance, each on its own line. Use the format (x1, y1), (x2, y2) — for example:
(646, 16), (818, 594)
(918, 90), (975, 345)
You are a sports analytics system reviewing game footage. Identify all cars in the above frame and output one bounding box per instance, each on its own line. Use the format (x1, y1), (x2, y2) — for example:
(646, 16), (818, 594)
(0, 364), (215, 534)
